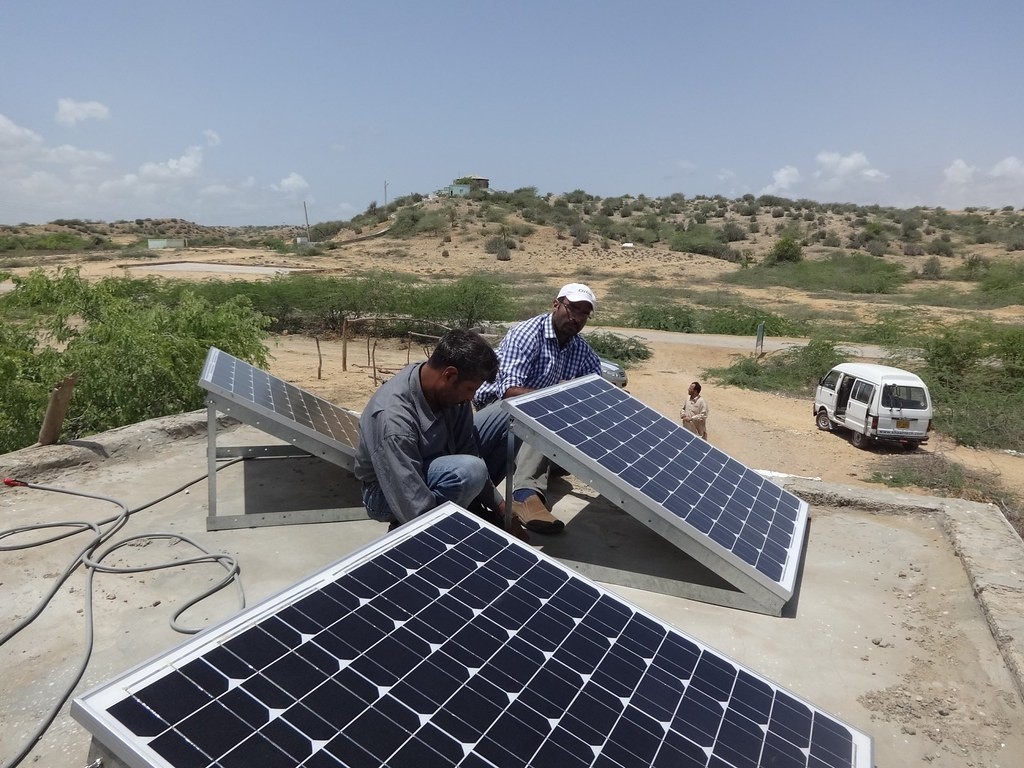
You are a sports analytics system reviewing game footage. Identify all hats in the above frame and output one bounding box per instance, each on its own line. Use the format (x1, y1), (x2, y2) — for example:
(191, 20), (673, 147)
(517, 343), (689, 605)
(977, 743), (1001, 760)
(557, 283), (596, 313)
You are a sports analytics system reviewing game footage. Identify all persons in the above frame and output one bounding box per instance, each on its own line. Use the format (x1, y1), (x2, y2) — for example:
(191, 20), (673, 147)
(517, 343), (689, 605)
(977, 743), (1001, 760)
(472, 283), (602, 536)
(679, 382), (708, 441)
(356, 329), (519, 534)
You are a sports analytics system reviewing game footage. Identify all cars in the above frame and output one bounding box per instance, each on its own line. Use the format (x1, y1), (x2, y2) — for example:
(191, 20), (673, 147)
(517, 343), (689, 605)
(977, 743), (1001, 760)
(598, 357), (629, 389)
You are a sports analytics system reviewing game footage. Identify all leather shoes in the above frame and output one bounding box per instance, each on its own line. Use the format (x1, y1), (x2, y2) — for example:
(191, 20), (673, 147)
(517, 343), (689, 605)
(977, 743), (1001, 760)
(511, 494), (565, 533)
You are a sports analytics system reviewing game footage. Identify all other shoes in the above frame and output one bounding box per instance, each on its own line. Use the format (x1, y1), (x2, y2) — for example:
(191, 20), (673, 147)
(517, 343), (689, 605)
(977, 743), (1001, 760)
(479, 510), (502, 528)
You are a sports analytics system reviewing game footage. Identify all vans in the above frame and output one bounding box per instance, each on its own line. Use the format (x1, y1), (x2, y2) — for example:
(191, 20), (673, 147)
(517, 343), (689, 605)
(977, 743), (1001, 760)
(812, 362), (934, 452)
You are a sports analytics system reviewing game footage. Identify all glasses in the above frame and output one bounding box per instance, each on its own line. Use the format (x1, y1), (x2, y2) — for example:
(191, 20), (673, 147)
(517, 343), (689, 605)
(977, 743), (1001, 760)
(560, 301), (592, 319)
